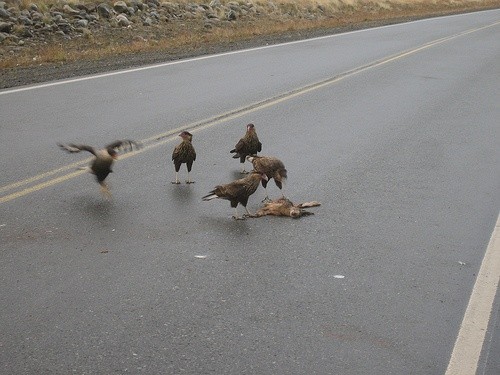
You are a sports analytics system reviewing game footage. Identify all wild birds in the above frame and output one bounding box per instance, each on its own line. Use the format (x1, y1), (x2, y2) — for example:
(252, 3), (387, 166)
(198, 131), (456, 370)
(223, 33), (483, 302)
(172, 130), (198, 185)
(201, 122), (321, 220)
(56, 138), (140, 197)
